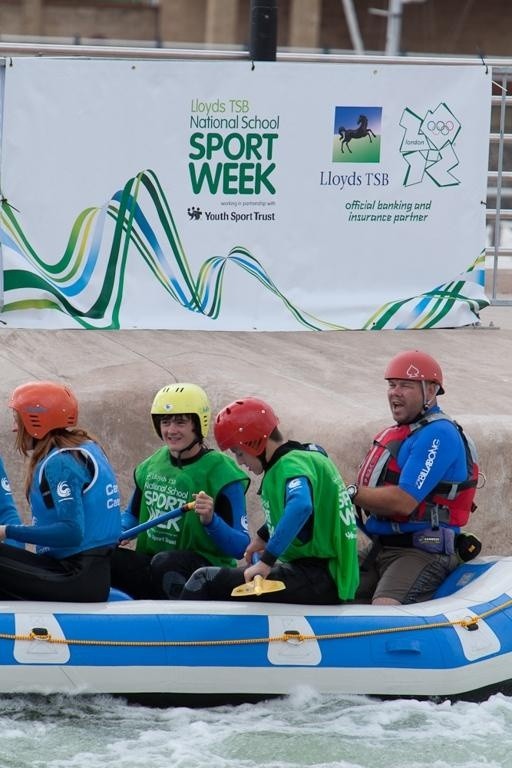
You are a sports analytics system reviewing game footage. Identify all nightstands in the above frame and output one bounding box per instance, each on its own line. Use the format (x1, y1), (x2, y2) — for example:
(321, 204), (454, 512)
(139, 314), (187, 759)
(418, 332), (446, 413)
(231, 550), (286, 597)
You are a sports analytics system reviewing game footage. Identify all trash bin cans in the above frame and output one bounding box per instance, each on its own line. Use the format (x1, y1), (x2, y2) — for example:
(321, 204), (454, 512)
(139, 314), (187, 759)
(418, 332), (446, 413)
(347, 481), (359, 502)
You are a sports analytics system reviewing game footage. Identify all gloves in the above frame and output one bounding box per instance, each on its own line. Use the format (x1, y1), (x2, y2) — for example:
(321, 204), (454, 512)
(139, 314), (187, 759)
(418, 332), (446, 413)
(0, 556), (512, 709)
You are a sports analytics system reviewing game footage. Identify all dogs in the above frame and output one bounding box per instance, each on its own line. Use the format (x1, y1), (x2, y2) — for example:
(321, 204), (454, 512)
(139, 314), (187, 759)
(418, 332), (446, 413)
(337, 114), (376, 154)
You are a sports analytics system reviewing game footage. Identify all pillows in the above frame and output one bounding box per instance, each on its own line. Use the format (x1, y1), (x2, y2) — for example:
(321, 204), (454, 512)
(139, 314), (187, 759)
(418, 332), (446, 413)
(213, 396), (281, 459)
(8, 379), (79, 440)
(382, 347), (446, 396)
(149, 381), (211, 442)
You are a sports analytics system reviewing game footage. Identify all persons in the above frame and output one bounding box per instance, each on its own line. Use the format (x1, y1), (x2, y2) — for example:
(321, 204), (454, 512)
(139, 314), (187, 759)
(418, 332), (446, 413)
(344, 349), (481, 605)
(180, 395), (363, 604)
(110, 382), (250, 600)
(1, 461), (27, 551)
(0, 380), (123, 603)
